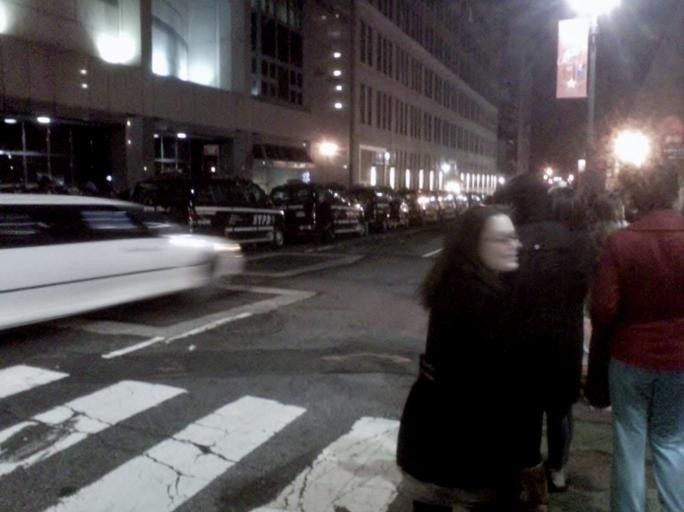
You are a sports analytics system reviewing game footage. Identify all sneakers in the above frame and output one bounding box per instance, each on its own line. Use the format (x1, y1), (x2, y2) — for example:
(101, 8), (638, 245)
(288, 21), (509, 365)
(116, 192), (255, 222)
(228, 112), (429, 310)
(551, 467), (567, 489)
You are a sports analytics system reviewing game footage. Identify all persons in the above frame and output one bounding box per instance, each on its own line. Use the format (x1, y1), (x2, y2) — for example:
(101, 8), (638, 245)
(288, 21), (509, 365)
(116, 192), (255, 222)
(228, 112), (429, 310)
(509, 164), (684, 512)
(391, 202), (553, 512)
(18, 173), (102, 197)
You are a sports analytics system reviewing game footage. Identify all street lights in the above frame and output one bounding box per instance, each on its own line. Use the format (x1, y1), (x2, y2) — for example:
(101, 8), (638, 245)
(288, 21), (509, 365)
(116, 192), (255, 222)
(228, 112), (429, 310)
(564, 1), (624, 167)
